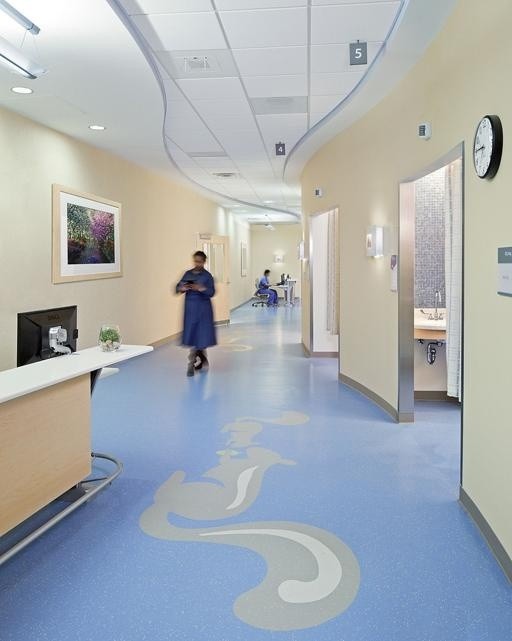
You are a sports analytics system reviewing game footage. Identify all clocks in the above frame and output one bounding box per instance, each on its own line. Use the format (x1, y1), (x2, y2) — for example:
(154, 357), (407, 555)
(473, 115), (502, 180)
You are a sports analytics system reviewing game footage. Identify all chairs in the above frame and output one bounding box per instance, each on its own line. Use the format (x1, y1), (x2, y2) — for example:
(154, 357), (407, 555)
(251, 278), (270, 307)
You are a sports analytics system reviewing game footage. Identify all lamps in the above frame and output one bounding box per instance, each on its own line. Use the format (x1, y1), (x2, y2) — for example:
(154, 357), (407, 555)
(0, 0), (50, 80)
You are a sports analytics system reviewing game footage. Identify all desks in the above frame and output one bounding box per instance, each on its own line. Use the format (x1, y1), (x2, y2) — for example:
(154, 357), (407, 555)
(270, 280), (297, 307)
(0, 343), (155, 570)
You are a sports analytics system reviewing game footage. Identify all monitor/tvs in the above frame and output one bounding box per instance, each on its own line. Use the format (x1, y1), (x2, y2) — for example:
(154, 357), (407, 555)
(17, 306), (78, 367)
(281, 273), (284, 284)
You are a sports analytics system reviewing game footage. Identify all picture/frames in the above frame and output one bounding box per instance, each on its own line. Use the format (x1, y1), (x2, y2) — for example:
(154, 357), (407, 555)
(241, 241), (248, 276)
(51, 182), (123, 285)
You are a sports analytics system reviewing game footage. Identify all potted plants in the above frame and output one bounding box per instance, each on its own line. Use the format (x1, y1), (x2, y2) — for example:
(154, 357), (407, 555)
(98, 323), (123, 352)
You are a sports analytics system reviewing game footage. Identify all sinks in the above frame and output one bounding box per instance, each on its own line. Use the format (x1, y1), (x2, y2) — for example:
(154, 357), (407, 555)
(414, 317), (446, 330)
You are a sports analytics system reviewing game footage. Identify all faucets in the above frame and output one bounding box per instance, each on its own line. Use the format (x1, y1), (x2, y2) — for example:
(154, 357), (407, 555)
(434, 289), (442, 317)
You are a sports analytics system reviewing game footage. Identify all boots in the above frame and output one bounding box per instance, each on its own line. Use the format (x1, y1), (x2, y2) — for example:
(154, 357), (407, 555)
(187, 351), (207, 376)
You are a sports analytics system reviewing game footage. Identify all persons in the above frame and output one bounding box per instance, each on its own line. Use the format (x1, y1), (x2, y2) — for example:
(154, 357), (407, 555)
(258, 270), (278, 306)
(176, 251), (218, 376)
